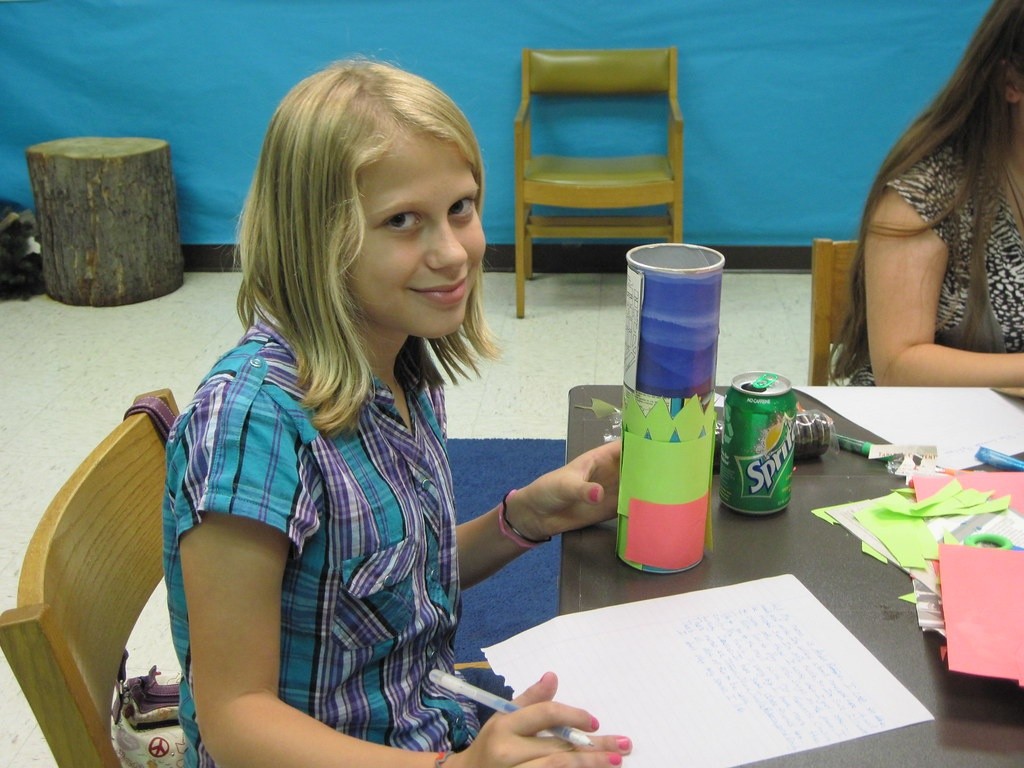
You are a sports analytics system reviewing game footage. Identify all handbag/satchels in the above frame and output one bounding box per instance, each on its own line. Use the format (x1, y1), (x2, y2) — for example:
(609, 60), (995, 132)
(110, 398), (186, 768)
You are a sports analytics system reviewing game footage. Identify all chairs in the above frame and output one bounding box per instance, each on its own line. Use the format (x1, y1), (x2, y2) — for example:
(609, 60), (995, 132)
(0, 381), (186, 768)
(808, 237), (863, 388)
(510, 46), (692, 318)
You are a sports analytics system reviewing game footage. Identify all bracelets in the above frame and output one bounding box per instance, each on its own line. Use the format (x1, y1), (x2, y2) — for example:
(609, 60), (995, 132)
(497, 489), (552, 548)
(435, 750), (454, 768)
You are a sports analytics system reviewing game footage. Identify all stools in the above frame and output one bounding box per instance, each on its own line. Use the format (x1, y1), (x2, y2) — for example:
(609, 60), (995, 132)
(23, 134), (191, 311)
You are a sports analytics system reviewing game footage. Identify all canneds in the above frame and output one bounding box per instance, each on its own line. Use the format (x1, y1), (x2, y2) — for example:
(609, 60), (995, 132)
(719, 371), (796, 515)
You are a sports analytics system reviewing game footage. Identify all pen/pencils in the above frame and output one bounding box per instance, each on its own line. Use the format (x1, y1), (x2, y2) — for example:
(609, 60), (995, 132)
(837, 433), (894, 461)
(428, 669), (595, 749)
(974, 446), (1024, 472)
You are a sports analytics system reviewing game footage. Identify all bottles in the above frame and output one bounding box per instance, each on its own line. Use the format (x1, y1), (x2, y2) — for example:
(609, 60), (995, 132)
(713, 409), (834, 468)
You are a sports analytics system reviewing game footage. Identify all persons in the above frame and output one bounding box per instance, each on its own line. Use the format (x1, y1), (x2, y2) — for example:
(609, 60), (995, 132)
(162, 60), (632, 768)
(830, 0), (1024, 388)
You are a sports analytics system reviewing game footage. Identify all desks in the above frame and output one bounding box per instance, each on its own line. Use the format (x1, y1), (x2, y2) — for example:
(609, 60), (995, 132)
(556, 373), (1024, 768)
